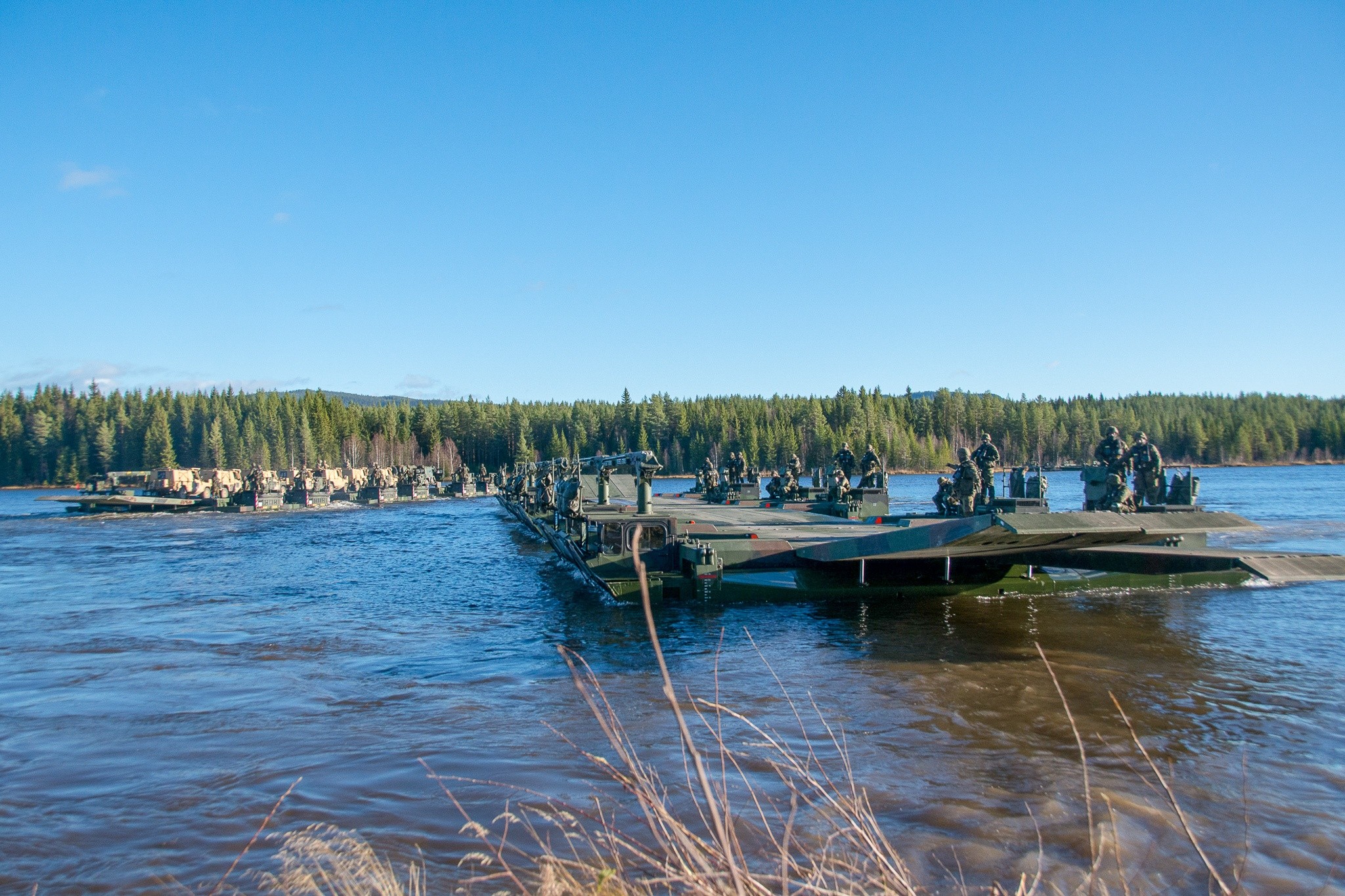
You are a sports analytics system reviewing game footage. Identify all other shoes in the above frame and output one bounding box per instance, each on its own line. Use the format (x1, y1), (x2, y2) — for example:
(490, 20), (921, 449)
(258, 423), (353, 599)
(707, 502), (710, 504)
(837, 499), (842, 502)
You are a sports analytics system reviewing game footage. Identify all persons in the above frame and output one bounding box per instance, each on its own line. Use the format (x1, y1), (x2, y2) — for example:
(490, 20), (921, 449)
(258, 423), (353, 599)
(294, 465), (308, 489)
(417, 476), (422, 486)
(110, 472), (120, 495)
(278, 476), (283, 483)
(247, 463), (256, 490)
(362, 464), (391, 488)
(479, 463), (486, 482)
(344, 459), (352, 468)
(695, 451), (802, 498)
(1096, 474), (1137, 513)
(315, 459), (329, 469)
(833, 441), (882, 502)
(253, 465), (263, 490)
(403, 464), (414, 484)
(238, 476), (251, 494)
(932, 433), (1000, 516)
(1110, 431), (1161, 506)
(211, 470), (221, 498)
(1094, 426), (1133, 485)
(458, 463), (469, 477)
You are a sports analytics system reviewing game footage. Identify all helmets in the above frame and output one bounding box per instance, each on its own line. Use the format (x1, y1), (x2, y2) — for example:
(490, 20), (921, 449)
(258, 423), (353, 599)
(1107, 474), (1122, 485)
(302, 465), (306, 470)
(866, 445), (872, 449)
(481, 464), (484, 467)
(957, 447), (970, 457)
(730, 452), (734, 456)
(707, 462), (713, 468)
(837, 470), (844, 475)
(737, 452), (742, 455)
(842, 442), (848, 447)
(785, 472), (791, 476)
(937, 476), (946, 484)
(318, 460), (327, 463)
(981, 433), (991, 440)
(463, 463), (466, 466)
(772, 471), (777, 475)
(1105, 425), (1119, 436)
(790, 454), (796, 458)
(252, 463), (261, 468)
(1133, 431), (1147, 441)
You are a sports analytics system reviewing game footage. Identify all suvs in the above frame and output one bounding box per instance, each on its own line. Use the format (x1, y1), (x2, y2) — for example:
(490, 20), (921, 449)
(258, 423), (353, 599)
(146, 469), (210, 499)
(248, 465), (443, 494)
(203, 469), (243, 498)
